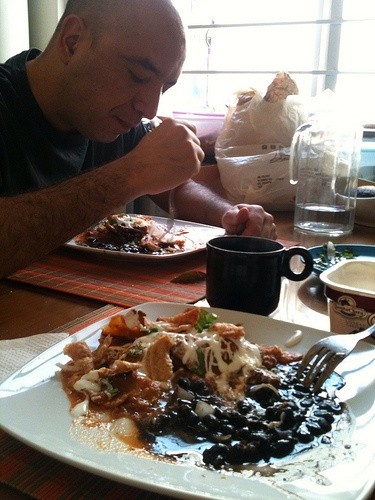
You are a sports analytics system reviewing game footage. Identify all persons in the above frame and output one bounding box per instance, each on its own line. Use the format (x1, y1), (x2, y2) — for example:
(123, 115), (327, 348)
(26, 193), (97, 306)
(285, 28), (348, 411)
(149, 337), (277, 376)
(0, 0), (276, 278)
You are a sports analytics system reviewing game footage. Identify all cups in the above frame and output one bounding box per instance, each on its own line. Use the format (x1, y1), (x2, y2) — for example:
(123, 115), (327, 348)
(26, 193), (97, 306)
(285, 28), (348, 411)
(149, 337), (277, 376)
(206, 235), (313, 317)
(288, 112), (363, 238)
(319, 258), (375, 346)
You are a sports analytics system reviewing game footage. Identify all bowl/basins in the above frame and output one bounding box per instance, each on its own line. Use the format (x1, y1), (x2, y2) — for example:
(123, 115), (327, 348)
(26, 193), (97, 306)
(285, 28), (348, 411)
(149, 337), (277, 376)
(335, 176), (375, 228)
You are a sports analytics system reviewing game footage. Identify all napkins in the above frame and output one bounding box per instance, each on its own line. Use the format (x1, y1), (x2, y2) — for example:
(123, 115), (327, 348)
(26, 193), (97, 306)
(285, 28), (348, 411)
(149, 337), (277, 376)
(0, 331), (69, 387)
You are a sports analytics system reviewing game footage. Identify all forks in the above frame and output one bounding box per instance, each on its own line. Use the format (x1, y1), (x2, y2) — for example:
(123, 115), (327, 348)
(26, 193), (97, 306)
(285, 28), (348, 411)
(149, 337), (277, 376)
(159, 188), (175, 240)
(296, 323), (375, 390)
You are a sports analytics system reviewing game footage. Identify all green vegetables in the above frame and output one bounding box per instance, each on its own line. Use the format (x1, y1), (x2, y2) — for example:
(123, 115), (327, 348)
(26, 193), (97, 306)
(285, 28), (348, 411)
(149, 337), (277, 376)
(100, 308), (219, 396)
(313, 241), (359, 270)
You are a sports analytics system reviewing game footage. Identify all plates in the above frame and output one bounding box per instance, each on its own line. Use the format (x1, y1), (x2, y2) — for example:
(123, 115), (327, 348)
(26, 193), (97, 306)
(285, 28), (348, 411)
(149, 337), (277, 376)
(301, 244), (375, 274)
(62, 213), (227, 264)
(0, 300), (375, 500)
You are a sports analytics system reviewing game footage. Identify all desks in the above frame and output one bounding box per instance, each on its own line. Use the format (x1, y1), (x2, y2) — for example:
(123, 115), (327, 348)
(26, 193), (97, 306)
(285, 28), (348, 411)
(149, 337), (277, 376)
(0, 209), (375, 500)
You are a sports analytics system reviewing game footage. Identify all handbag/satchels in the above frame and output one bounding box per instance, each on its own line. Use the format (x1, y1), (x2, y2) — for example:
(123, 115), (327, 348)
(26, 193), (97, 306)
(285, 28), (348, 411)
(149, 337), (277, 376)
(214, 72), (308, 214)
